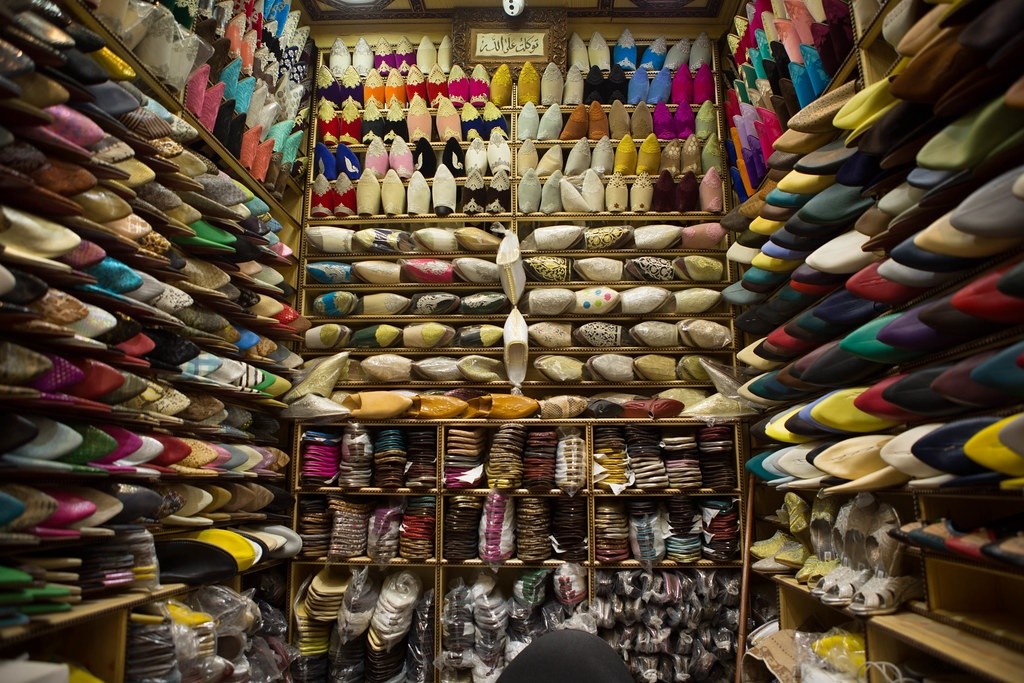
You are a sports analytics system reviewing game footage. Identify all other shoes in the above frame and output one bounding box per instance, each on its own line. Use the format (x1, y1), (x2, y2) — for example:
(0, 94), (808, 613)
(2, 0), (1024, 683)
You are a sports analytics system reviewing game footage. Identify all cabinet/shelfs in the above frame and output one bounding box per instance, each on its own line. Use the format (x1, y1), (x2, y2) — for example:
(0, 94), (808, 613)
(0, 0), (1024, 683)
